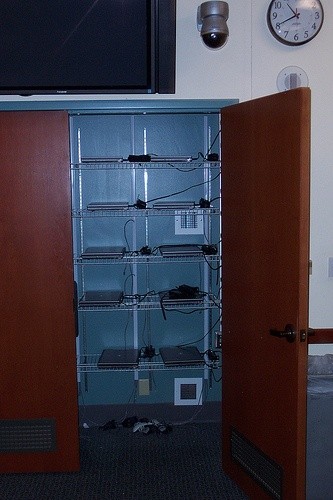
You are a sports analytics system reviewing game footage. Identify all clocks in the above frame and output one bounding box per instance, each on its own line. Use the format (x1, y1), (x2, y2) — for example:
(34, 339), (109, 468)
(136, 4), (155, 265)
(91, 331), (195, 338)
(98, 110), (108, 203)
(267, 0), (324, 46)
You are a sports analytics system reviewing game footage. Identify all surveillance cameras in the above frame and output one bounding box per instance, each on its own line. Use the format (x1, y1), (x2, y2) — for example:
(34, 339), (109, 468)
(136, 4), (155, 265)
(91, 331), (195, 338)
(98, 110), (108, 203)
(197, 0), (229, 48)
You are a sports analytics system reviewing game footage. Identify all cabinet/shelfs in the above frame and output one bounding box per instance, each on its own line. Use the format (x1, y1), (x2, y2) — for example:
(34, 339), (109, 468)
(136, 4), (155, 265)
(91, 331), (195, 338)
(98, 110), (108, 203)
(69, 111), (220, 383)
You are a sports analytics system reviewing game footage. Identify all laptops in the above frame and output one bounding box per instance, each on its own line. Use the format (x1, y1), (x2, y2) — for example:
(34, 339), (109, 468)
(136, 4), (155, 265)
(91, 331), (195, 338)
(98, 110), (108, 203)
(86, 202), (130, 210)
(81, 157), (124, 162)
(153, 201), (195, 209)
(159, 346), (205, 367)
(160, 246), (203, 257)
(97, 348), (139, 368)
(82, 247), (126, 258)
(150, 155), (192, 162)
(78, 291), (125, 306)
(158, 291), (204, 306)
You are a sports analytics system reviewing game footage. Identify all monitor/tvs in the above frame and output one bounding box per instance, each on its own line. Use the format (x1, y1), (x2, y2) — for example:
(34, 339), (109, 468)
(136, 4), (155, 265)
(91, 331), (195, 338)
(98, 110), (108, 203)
(0, 0), (177, 95)
